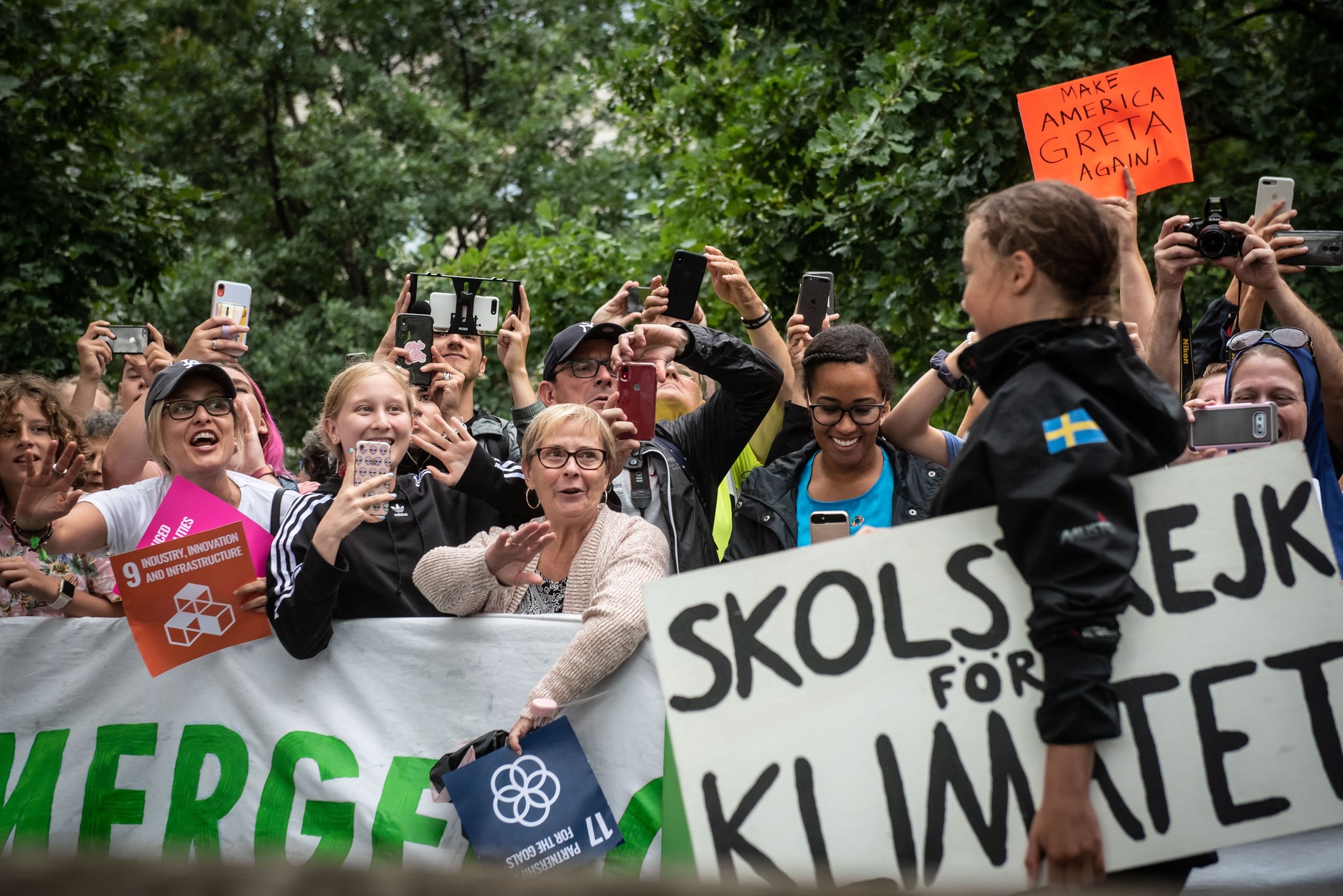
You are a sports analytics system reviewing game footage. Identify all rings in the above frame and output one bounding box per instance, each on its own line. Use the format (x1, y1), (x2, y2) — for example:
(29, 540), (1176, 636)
(169, 361), (172, 366)
(443, 372), (446, 380)
(52, 463), (67, 477)
(727, 263), (734, 275)
(445, 373), (451, 380)
(213, 339), (217, 350)
(396, 365), (401, 372)
(510, 532), (518, 546)
(1124, 201), (1129, 207)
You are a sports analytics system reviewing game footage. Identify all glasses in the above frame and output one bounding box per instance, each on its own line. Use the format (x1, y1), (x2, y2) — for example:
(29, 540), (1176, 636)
(157, 394), (237, 422)
(805, 383), (888, 427)
(657, 363), (702, 387)
(529, 446), (609, 471)
(1226, 326), (1322, 390)
(553, 357), (620, 379)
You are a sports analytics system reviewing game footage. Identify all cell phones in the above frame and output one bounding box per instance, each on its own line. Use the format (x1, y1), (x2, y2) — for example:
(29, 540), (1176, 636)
(808, 510), (851, 545)
(430, 292), (499, 332)
(660, 250), (707, 321)
(395, 313), (434, 386)
(355, 441), (390, 516)
(796, 274), (830, 338)
(1187, 401), (1279, 452)
(625, 287), (651, 313)
(805, 271), (835, 328)
(615, 361), (658, 442)
(1273, 231), (1343, 268)
(1253, 176), (1295, 226)
(96, 324), (151, 354)
(210, 280), (252, 356)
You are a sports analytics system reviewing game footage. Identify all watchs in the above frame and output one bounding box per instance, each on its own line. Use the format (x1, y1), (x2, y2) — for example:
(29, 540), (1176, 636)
(740, 303), (772, 329)
(250, 463), (277, 479)
(46, 579), (75, 610)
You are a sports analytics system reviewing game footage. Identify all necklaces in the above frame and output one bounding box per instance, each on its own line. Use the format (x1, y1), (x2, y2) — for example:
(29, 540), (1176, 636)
(229, 485), (232, 504)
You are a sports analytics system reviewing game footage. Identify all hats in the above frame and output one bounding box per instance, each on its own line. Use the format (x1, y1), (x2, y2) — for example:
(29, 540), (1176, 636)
(143, 359), (238, 421)
(542, 321), (629, 382)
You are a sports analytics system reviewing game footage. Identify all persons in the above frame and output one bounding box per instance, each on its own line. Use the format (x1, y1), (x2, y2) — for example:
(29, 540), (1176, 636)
(0, 245), (989, 659)
(1095, 166), (1343, 581)
(411, 404), (670, 755)
(929, 179), (1191, 896)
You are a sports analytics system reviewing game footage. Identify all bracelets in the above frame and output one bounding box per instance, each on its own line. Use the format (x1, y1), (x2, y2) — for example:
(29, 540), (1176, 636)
(10, 517), (55, 552)
(454, 416), (464, 434)
(930, 349), (974, 392)
(967, 331), (976, 346)
(173, 360), (175, 364)
(15, 523), (50, 538)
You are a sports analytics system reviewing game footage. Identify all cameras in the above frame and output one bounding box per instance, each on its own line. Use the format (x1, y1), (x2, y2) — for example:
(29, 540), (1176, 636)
(1173, 197), (1245, 260)
